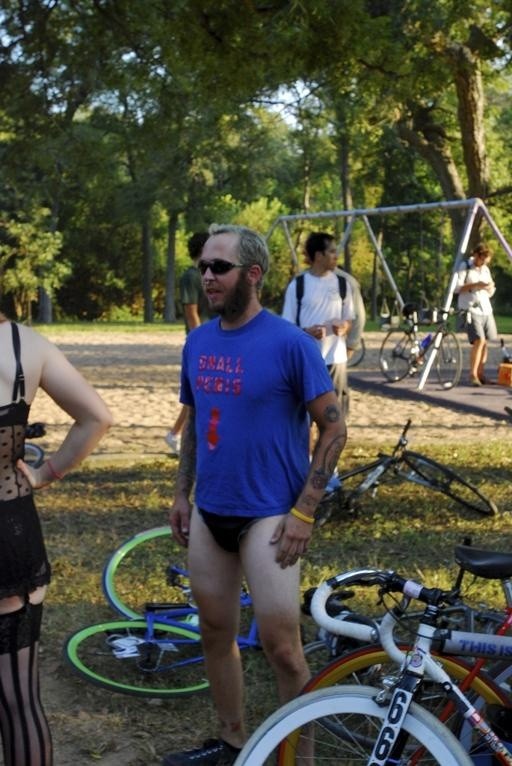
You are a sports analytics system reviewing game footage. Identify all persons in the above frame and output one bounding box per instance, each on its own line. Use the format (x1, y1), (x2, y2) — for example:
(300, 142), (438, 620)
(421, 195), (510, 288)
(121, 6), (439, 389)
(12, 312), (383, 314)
(162, 228), (221, 457)
(280, 229), (356, 503)
(163, 220), (342, 764)
(450, 239), (501, 388)
(302, 229), (368, 362)
(0, 287), (117, 764)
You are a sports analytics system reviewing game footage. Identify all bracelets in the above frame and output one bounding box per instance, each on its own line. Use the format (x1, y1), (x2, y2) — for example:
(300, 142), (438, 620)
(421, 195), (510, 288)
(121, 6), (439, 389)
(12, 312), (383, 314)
(45, 459), (64, 483)
(289, 506), (316, 526)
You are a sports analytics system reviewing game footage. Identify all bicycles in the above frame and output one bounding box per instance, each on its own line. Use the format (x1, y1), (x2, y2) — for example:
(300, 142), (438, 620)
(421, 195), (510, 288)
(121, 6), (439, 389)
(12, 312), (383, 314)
(311, 419), (497, 529)
(62, 524), (303, 699)
(379, 304), (464, 389)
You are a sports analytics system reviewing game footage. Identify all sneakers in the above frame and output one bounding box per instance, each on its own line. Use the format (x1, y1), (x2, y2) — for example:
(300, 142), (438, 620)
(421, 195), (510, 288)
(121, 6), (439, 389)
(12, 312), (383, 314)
(165, 431), (180, 454)
(160, 738), (242, 766)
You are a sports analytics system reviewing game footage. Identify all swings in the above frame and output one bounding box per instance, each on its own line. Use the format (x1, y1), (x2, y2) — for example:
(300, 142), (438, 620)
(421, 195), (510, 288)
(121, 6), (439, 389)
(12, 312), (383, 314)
(376, 203), (443, 329)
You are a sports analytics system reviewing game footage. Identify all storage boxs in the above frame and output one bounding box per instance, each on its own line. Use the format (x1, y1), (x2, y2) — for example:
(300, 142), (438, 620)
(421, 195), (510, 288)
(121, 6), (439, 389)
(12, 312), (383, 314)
(496, 363), (512, 386)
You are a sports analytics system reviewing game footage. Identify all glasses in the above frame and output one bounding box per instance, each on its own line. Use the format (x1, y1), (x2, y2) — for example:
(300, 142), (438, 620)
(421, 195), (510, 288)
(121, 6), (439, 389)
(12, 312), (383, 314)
(198, 258), (245, 276)
